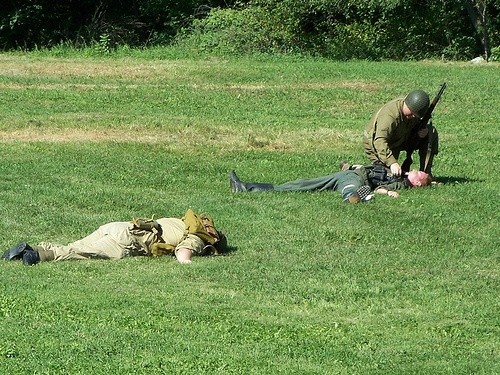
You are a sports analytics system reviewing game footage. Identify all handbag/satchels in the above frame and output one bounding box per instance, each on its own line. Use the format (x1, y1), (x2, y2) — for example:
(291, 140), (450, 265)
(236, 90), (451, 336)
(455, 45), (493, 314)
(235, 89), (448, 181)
(182, 208), (220, 245)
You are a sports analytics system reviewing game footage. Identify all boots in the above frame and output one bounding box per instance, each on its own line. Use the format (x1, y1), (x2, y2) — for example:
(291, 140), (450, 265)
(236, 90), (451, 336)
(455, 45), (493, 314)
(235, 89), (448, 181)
(342, 183), (372, 204)
(2, 241), (43, 261)
(227, 169), (274, 193)
(419, 150), (445, 186)
(22, 247), (54, 267)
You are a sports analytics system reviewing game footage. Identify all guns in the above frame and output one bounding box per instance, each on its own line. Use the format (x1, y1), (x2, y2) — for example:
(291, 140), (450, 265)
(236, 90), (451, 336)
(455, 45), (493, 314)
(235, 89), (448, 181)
(401, 83), (446, 173)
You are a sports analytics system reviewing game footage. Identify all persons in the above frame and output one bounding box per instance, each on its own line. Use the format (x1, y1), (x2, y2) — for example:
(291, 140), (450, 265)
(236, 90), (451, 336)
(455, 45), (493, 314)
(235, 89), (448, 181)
(230, 163), (431, 203)
(366, 90), (440, 175)
(1, 208), (229, 266)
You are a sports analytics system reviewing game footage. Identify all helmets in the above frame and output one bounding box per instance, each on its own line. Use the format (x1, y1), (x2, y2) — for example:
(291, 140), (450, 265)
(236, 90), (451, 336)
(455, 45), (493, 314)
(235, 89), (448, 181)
(404, 90), (428, 120)
